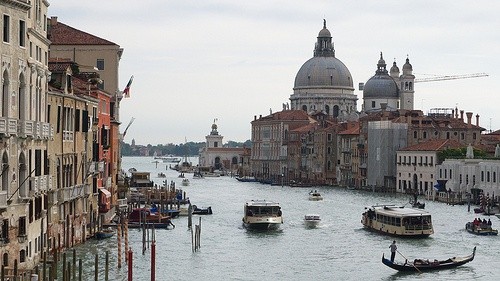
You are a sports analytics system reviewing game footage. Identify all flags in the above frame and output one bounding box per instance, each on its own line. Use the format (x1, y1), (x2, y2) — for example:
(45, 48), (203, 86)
(124, 79), (131, 98)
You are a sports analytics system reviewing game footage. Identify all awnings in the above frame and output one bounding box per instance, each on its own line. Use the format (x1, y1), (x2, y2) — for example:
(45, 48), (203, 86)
(100, 189), (111, 198)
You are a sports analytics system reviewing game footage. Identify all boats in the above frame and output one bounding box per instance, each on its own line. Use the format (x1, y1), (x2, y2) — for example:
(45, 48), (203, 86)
(242, 197), (284, 230)
(413, 203), (426, 209)
(304, 215), (322, 227)
(182, 179), (191, 186)
(150, 206), (213, 218)
(362, 205), (434, 238)
(381, 246), (477, 271)
(194, 171), (225, 177)
(113, 208), (171, 227)
(309, 193), (323, 201)
(237, 177), (260, 182)
(466, 222), (498, 236)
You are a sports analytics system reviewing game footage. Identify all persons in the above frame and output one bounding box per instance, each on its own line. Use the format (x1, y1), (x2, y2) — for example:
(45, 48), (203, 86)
(389, 241), (397, 262)
(474, 218), (491, 225)
